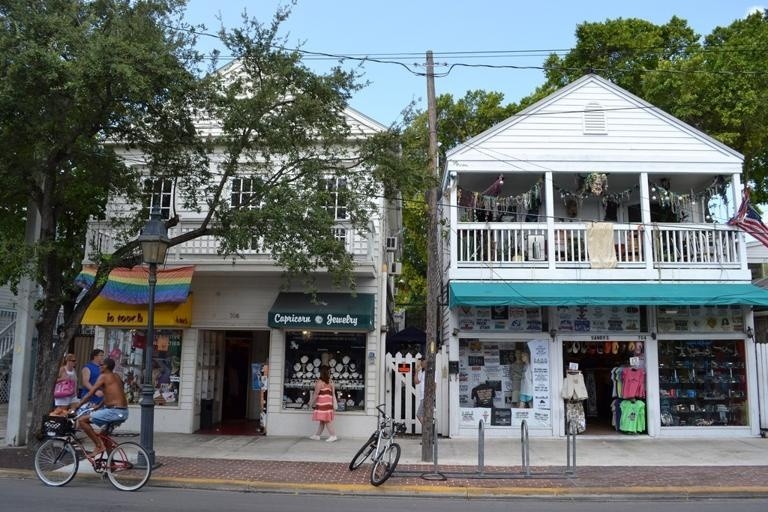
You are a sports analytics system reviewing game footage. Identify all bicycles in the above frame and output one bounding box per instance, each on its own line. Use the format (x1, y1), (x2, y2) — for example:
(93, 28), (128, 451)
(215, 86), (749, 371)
(347, 401), (408, 487)
(33, 406), (153, 491)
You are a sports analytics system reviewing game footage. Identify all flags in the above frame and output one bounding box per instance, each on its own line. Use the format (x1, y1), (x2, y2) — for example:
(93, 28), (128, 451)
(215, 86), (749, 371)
(726, 187), (767, 249)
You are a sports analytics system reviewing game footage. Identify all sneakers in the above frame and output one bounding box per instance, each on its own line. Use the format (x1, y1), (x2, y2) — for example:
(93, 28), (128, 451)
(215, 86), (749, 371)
(326, 436), (337, 441)
(310, 434), (320, 440)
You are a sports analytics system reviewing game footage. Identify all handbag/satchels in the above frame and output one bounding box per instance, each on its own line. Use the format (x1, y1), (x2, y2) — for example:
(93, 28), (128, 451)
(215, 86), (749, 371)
(53, 380), (75, 397)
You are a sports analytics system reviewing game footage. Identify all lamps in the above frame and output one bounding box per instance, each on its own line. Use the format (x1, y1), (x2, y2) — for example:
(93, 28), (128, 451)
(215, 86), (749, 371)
(368, 352), (376, 364)
(394, 279), (405, 285)
(551, 328), (556, 336)
(452, 328), (459, 336)
(746, 326), (754, 338)
(664, 308), (679, 314)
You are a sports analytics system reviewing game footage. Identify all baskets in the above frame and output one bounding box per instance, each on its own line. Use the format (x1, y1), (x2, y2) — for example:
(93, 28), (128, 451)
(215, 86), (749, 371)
(41, 415), (67, 435)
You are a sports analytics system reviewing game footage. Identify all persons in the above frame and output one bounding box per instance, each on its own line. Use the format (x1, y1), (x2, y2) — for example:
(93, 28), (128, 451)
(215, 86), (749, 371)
(305, 365), (337, 443)
(68, 356), (129, 477)
(414, 353), (437, 447)
(78, 348), (107, 415)
(257, 364), (268, 435)
(554, 218), (571, 255)
(517, 351), (531, 409)
(53, 353), (82, 411)
(509, 349), (523, 409)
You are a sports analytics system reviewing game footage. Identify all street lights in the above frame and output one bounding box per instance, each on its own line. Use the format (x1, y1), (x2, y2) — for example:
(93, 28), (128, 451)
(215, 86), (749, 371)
(130, 216), (174, 472)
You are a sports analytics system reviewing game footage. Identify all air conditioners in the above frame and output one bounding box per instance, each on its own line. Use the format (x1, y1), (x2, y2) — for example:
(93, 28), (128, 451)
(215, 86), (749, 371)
(386, 237), (398, 251)
(389, 262), (397, 273)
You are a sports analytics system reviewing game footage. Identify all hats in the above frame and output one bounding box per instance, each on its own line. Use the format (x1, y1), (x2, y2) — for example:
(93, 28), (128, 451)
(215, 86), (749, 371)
(418, 355), (425, 360)
(566, 341), (643, 354)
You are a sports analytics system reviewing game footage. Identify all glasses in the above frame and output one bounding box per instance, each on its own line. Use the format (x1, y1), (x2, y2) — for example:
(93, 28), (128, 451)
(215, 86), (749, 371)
(69, 359), (76, 362)
